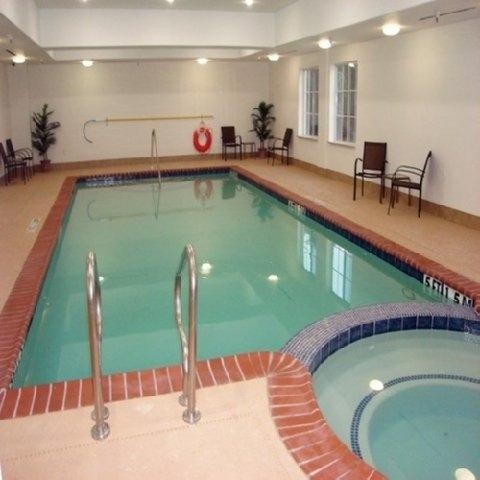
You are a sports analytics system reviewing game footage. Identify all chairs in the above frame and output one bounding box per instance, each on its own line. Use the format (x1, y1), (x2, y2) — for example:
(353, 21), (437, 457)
(0, 138), (34, 185)
(267, 127), (293, 166)
(352, 141), (431, 218)
(221, 125), (241, 162)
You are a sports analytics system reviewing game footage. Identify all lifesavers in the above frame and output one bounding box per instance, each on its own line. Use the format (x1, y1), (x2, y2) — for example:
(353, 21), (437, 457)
(194, 178), (213, 200)
(194, 127), (211, 152)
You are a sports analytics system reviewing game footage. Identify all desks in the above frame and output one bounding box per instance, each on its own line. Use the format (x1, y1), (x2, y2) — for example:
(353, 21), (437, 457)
(242, 141), (256, 159)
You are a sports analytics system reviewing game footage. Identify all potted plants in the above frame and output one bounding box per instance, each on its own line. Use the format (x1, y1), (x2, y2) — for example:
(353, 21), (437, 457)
(251, 101), (275, 159)
(31, 103), (61, 171)
